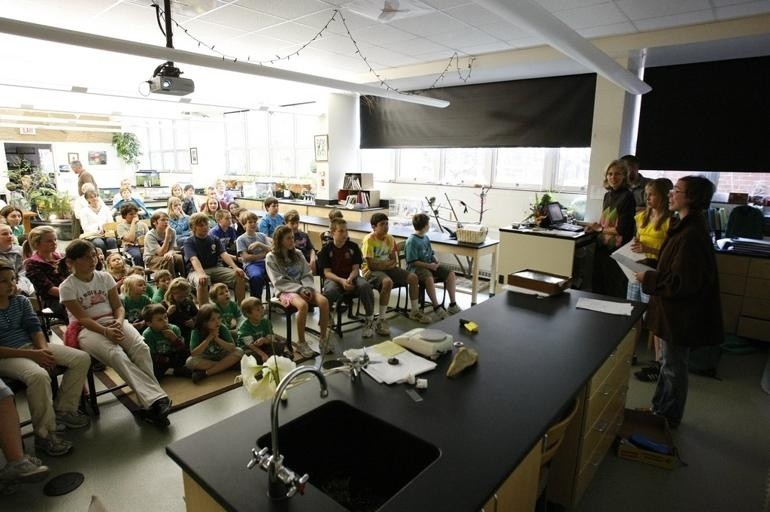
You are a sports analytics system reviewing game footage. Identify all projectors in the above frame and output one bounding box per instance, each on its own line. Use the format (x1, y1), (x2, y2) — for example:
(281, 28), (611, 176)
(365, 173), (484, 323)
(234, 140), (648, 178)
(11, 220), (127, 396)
(150, 76), (195, 96)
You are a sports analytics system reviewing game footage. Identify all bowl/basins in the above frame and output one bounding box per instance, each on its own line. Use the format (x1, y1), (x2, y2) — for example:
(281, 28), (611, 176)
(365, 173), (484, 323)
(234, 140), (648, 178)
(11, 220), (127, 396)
(541, 396), (579, 465)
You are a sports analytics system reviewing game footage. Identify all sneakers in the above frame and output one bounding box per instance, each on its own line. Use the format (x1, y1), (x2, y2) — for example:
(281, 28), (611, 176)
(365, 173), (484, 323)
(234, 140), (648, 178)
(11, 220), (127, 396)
(139, 408), (171, 427)
(266, 301), (287, 315)
(0, 453), (50, 499)
(319, 337), (335, 354)
(53, 410), (91, 429)
(361, 319), (374, 339)
(408, 309), (433, 324)
(623, 406), (683, 427)
(151, 396), (173, 422)
(434, 306), (449, 320)
(447, 303), (464, 314)
(630, 351), (663, 382)
(295, 340), (314, 358)
(33, 436), (75, 457)
(191, 369), (208, 383)
(171, 366), (188, 377)
(375, 317), (390, 335)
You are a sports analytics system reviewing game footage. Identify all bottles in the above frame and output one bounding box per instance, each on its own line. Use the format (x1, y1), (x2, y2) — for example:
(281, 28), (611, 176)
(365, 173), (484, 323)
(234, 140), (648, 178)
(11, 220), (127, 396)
(144, 179), (151, 188)
(284, 189), (290, 198)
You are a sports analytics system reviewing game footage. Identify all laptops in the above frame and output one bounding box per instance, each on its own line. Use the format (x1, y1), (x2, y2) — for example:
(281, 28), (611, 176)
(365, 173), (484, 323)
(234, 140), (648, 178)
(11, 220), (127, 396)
(545, 202), (583, 232)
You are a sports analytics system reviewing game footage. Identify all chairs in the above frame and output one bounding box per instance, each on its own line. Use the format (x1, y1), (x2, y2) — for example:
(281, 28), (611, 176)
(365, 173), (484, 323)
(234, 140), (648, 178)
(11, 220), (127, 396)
(84, 353), (130, 415)
(1, 373), (88, 450)
(319, 269), (366, 339)
(180, 246), (192, 277)
(535, 397), (581, 504)
(265, 276), (316, 352)
(372, 282), (409, 321)
(33, 283), (69, 343)
(395, 241), (447, 311)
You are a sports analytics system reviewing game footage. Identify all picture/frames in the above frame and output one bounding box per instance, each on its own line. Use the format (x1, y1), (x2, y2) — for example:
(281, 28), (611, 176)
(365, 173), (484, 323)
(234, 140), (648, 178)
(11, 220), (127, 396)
(189, 146), (199, 166)
(67, 152), (80, 165)
(313, 133), (330, 162)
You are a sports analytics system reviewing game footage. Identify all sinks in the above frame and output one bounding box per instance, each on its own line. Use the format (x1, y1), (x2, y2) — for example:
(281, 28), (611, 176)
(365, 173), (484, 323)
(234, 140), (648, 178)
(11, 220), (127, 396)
(256, 398), (442, 512)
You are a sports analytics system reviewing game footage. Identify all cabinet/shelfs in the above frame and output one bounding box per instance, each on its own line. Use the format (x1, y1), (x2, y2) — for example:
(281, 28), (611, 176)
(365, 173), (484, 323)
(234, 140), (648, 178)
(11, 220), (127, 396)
(549, 319), (637, 511)
(703, 242), (770, 347)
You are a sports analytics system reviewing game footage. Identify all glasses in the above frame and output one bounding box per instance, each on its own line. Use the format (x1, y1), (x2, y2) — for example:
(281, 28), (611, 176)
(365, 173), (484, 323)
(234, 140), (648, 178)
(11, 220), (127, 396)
(668, 189), (690, 194)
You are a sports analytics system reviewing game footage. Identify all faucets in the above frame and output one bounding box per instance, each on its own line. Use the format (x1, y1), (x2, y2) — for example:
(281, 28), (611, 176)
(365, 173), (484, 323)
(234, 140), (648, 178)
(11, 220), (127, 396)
(265, 367), (328, 501)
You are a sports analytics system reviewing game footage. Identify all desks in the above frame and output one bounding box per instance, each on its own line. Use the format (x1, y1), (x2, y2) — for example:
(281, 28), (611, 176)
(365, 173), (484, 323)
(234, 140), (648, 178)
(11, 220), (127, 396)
(497, 222), (599, 290)
(247, 207), (500, 307)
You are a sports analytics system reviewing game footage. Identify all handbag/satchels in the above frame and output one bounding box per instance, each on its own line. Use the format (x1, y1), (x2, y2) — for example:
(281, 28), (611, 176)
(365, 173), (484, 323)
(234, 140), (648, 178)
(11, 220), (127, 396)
(725, 205), (765, 241)
